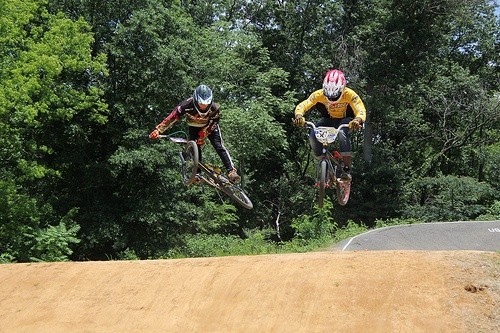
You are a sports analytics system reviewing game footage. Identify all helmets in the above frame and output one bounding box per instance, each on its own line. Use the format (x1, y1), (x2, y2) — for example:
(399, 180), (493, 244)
(193, 85), (213, 117)
(323, 69), (346, 103)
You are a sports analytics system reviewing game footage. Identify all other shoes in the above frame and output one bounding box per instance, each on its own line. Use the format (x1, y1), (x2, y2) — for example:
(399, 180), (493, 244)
(228, 169), (240, 181)
(341, 166), (352, 180)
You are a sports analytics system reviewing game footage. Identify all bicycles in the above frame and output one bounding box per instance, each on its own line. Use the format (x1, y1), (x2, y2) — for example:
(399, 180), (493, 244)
(142, 134), (253, 209)
(293, 119), (363, 208)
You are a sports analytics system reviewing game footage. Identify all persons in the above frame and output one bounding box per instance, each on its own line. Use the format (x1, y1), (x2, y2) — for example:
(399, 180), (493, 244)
(150, 84), (241, 184)
(293, 70), (367, 182)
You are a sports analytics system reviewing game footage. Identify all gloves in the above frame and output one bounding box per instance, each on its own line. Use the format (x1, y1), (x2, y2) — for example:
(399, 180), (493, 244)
(349, 118), (363, 129)
(149, 129), (159, 140)
(293, 116), (305, 127)
(196, 137), (205, 144)
